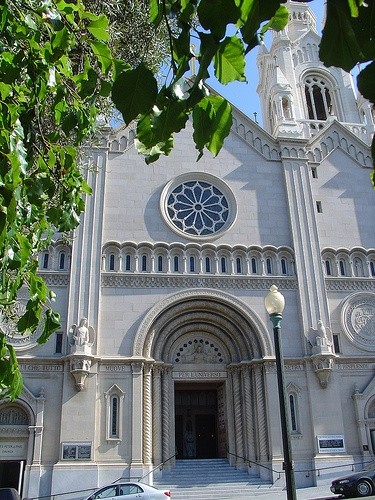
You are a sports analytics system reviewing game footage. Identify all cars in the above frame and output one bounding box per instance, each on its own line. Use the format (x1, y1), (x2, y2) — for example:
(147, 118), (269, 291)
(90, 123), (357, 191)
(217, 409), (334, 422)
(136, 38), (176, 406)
(84, 482), (171, 500)
(330, 459), (375, 497)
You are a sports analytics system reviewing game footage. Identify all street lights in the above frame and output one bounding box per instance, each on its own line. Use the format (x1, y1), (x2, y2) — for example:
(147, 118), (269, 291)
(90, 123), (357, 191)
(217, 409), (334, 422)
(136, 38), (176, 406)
(263, 284), (297, 500)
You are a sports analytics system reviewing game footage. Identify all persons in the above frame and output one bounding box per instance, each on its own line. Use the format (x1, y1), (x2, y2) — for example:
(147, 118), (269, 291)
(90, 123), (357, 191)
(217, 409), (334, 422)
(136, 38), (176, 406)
(72, 317), (90, 346)
